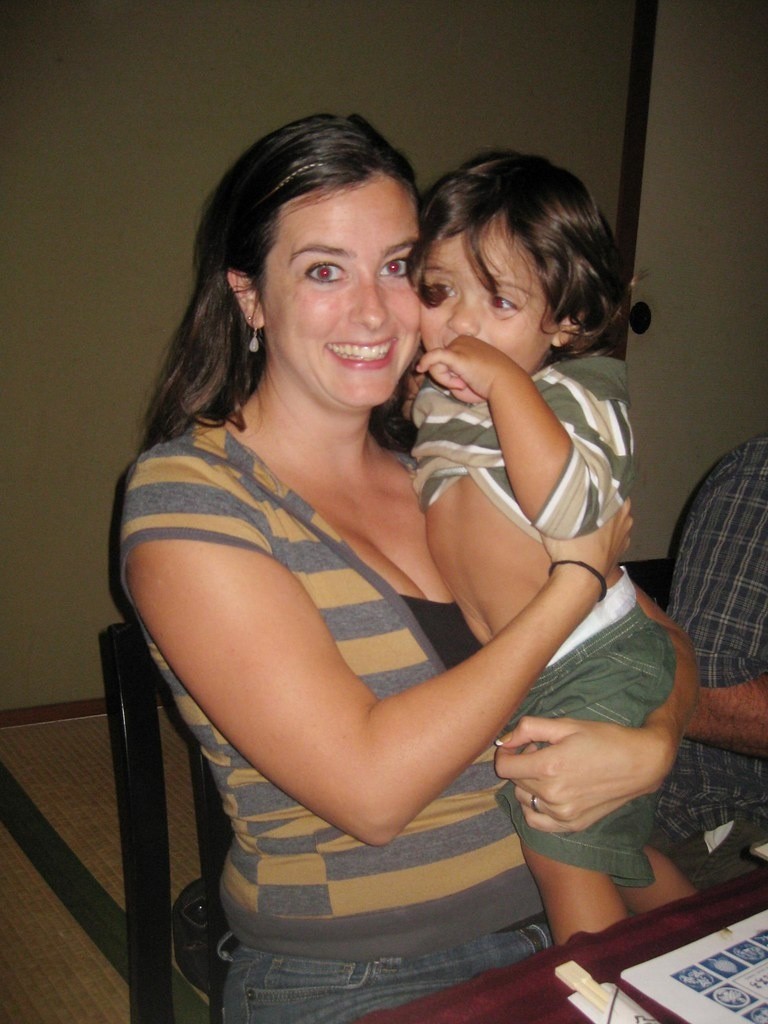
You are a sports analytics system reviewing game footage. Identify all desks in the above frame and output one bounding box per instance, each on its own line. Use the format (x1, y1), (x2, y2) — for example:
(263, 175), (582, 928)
(357, 865), (768, 1024)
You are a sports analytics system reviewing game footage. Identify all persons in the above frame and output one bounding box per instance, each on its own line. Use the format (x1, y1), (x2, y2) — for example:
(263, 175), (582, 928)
(120, 115), (697, 1024)
(649, 436), (768, 892)
(401, 152), (700, 954)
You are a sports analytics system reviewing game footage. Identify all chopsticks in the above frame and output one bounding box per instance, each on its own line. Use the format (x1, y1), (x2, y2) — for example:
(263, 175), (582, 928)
(554, 961), (610, 1015)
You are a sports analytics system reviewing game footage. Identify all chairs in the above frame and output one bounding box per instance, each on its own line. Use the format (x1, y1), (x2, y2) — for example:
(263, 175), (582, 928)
(106, 619), (236, 1024)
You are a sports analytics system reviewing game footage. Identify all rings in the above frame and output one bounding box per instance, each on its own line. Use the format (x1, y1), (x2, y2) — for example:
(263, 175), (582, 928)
(529, 796), (542, 814)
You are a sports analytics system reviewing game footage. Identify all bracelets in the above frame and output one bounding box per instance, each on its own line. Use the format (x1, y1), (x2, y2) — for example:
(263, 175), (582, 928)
(549, 560), (608, 603)
